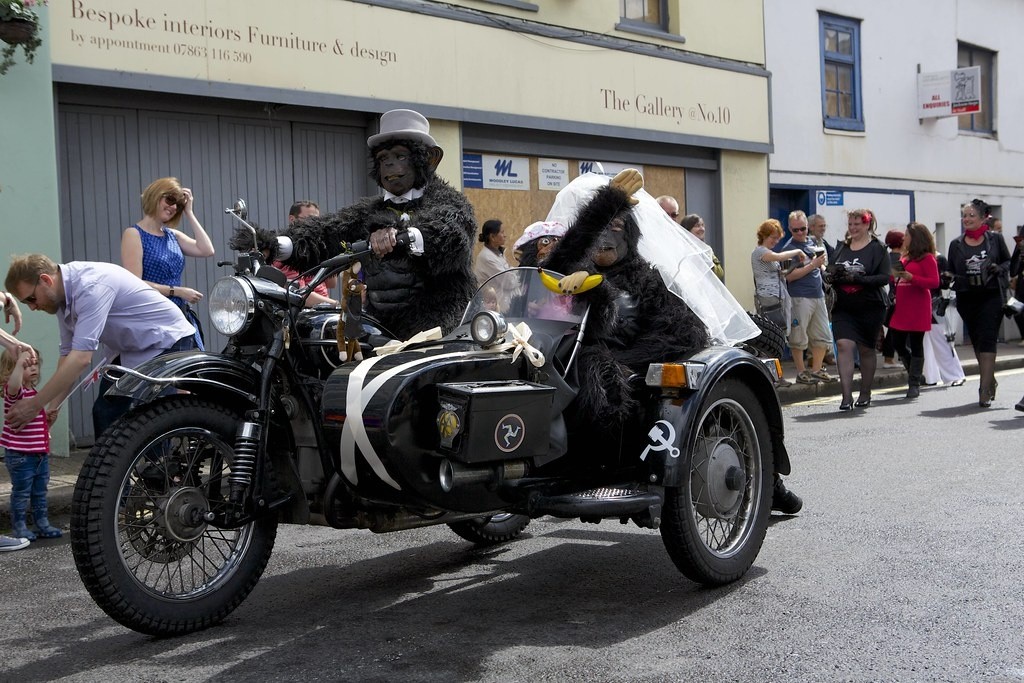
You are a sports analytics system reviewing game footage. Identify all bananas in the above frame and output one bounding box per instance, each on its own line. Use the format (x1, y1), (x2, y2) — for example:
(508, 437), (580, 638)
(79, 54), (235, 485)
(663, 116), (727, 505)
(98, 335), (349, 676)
(537, 267), (603, 294)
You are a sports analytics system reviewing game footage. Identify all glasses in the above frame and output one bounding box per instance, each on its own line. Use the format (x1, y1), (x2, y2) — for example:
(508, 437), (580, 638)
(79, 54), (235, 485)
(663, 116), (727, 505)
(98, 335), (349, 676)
(162, 194), (184, 215)
(667, 212), (679, 218)
(21, 272), (48, 305)
(792, 227), (806, 233)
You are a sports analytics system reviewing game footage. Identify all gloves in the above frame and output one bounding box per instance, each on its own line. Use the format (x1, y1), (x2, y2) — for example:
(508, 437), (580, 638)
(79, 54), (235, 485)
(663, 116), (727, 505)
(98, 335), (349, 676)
(987, 262), (1004, 274)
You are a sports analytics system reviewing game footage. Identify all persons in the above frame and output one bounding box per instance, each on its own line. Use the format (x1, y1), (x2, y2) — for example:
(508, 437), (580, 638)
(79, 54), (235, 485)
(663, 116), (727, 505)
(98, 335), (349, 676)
(680, 214), (724, 280)
(0, 290), (36, 372)
(942, 199), (1024, 407)
(822, 209), (892, 410)
(233, 109), (483, 344)
(4, 253), (196, 519)
(475, 219), (522, 317)
(120, 177), (215, 351)
(781, 210), (838, 385)
(655, 195), (679, 221)
(751, 218), (807, 389)
(808, 214), (835, 367)
(884, 222), (966, 398)
(273, 201), (340, 308)
(0, 349), (63, 540)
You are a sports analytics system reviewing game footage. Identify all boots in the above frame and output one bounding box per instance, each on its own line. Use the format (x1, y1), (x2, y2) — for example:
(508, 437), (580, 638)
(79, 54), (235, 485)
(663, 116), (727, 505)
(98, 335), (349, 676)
(30, 506), (63, 537)
(771, 473), (803, 514)
(10, 509), (36, 541)
(900, 355), (924, 398)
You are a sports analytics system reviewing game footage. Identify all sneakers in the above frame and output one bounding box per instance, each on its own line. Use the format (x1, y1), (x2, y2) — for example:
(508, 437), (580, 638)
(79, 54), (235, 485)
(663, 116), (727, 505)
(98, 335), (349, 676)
(811, 367), (831, 382)
(796, 371), (819, 384)
(0, 535), (30, 551)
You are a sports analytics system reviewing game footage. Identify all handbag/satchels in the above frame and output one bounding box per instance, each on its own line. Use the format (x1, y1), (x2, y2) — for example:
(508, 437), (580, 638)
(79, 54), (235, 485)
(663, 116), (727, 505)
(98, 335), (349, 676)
(1004, 296), (1024, 320)
(882, 302), (895, 327)
(763, 309), (787, 330)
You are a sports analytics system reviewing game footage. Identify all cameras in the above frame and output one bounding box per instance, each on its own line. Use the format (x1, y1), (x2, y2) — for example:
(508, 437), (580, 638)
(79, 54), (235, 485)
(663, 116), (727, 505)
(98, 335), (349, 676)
(936, 299), (950, 317)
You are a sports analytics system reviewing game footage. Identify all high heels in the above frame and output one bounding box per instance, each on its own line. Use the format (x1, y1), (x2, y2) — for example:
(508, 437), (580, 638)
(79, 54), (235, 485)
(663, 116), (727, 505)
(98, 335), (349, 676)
(979, 388), (991, 407)
(991, 379), (996, 400)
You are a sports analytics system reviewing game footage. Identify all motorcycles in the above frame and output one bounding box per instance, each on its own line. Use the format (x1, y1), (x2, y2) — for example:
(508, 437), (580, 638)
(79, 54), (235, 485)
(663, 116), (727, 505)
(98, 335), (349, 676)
(68, 197), (792, 636)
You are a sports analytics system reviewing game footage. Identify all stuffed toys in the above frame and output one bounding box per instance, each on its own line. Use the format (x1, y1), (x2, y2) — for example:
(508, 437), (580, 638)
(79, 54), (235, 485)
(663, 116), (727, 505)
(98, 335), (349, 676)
(512, 169), (706, 484)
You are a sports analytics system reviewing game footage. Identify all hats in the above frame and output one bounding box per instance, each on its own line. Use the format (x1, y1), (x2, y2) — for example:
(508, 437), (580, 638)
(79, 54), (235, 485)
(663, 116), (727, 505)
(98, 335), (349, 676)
(513, 221), (567, 250)
(366, 108), (439, 149)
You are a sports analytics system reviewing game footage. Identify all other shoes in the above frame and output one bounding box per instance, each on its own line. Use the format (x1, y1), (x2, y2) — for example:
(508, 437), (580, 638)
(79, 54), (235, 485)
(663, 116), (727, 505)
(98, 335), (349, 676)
(855, 398), (870, 407)
(808, 358), (813, 366)
(952, 378), (966, 386)
(840, 399), (853, 409)
(1015, 397), (1024, 412)
(823, 355), (836, 365)
(924, 383), (937, 385)
(1019, 340), (1024, 346)
(883, 362), (904, 369)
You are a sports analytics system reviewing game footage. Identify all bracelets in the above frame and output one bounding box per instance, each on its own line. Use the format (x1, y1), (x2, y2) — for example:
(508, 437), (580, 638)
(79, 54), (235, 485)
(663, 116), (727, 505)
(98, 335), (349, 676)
(3, 291), (12, 311)
(169, 285), (174, 296)
(810, 263), (815, 269)
